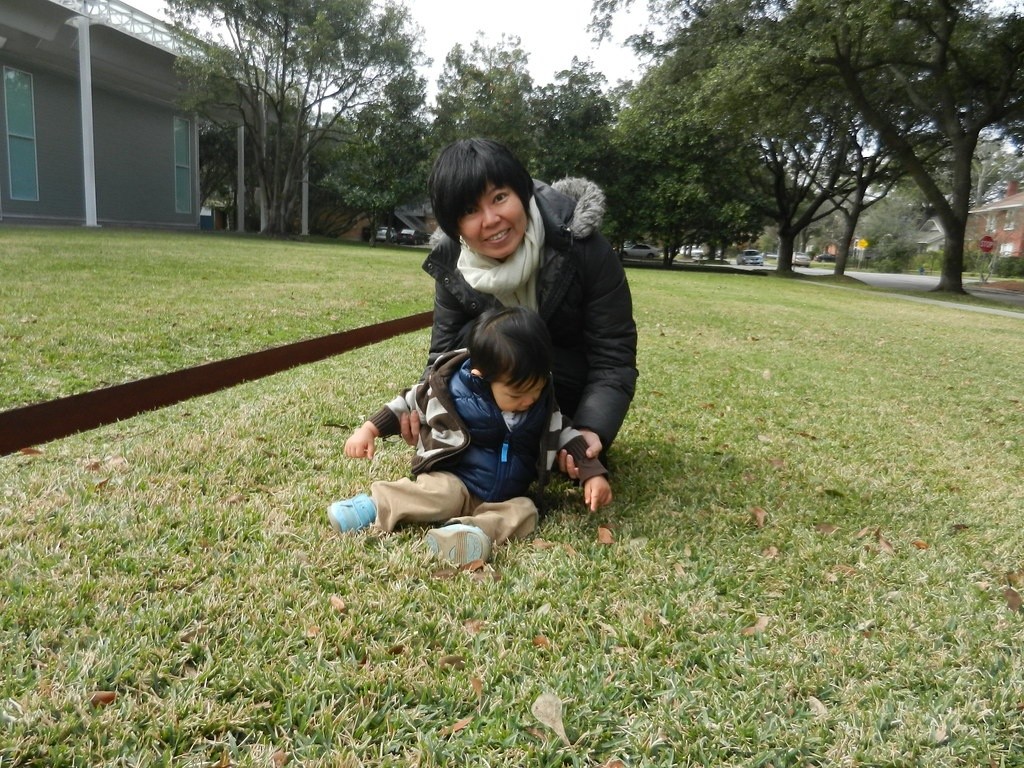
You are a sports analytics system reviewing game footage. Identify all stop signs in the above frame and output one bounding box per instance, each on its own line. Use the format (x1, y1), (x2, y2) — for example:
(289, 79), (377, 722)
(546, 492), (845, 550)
(980, 235), (994, 252)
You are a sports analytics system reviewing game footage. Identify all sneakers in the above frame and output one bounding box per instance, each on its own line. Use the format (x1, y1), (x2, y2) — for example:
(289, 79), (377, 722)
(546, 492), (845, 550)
(426, 524), (492, 568)
(327, 494), (376, 535)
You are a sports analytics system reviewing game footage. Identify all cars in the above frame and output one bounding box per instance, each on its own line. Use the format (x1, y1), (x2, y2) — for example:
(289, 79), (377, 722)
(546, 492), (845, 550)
(614, 243), (661, 259)
(735, 248), (764, 266)
(395, 228), (426, 246)
(791, 251), (811, 267)
(678, 245), (706, 260)
(814, 253), (836, 263)
(374, 226), (399, 244)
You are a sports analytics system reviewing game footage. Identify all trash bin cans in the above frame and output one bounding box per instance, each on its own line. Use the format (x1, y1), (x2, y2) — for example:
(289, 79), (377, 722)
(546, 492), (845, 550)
(362, 226), (370, 242)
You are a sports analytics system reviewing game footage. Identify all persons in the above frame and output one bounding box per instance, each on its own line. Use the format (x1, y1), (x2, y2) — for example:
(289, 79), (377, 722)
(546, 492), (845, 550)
(400, 138), (639, 478)
(326, 301), (614, 568)
